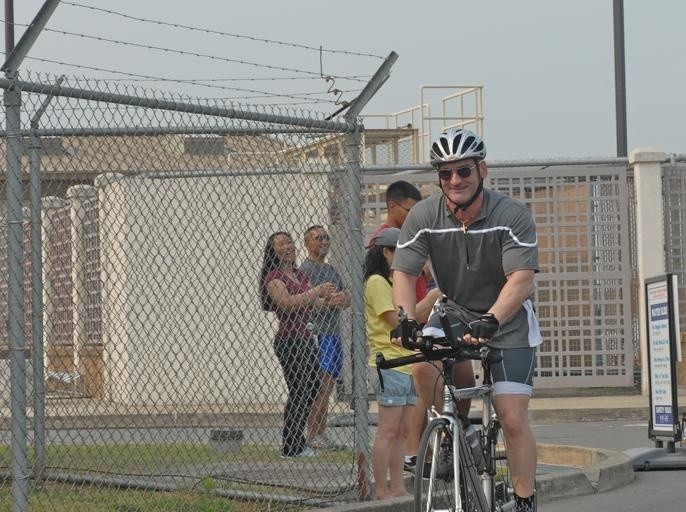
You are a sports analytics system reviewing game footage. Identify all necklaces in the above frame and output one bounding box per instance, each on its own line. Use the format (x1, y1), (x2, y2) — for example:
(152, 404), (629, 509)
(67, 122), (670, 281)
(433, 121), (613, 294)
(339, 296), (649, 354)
(450, 211), (482, 235)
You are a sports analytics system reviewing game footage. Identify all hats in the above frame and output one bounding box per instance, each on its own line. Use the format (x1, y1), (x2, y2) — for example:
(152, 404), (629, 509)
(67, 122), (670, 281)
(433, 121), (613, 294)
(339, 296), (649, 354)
(375, 227), (401, 246)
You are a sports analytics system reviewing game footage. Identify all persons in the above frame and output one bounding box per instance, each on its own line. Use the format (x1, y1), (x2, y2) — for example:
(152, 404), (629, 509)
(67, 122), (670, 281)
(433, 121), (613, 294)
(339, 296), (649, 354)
(388, 126), (544, 512)
(362, 227), (444, 503)
(421, 254), (476, 480)
(298, 224), (352, 452)
(368, 180), (428, 475)
(258, 231), (337, 459)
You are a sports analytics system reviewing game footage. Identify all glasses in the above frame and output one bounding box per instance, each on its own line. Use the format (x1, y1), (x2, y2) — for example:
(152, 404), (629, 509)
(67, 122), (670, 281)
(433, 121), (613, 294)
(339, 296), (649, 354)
(315, 235), (330, 241)
(439, 160), (484, 180)
(388, 200), (410, 212)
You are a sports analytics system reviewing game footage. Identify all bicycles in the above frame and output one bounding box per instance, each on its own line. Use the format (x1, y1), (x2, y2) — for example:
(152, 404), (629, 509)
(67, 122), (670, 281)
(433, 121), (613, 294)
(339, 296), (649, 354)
(375, 292), (539, 512)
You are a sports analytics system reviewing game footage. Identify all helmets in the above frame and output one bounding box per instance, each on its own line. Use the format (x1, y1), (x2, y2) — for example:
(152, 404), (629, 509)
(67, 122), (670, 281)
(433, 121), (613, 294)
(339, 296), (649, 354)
(430, 128), (486, 165)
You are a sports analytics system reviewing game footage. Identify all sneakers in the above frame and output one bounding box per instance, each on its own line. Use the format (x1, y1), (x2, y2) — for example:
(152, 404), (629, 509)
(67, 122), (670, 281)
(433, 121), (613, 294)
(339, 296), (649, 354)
(512, 493), (534, 512)
(404, 455), (431, 481)
(436, 437), (454, 479)
(305, 435), (347, 452)
(281, 447), (320, 459)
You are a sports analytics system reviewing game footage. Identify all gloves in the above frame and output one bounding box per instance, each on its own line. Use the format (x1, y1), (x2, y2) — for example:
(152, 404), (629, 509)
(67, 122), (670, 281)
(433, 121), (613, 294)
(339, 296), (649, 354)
(464, 313), (499, 339)
(391, 319), (423, 338)
